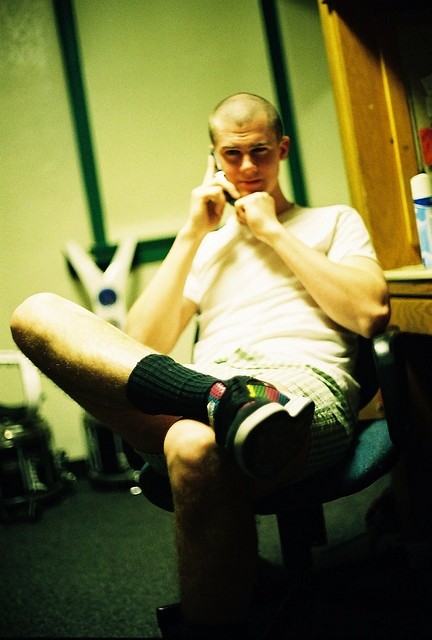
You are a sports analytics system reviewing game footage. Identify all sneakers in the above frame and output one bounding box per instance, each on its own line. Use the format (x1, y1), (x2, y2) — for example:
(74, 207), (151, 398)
(213, 377), (314, 517)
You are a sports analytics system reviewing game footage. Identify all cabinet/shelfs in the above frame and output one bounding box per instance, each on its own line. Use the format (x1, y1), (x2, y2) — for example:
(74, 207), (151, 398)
(315, 0), (432, 422)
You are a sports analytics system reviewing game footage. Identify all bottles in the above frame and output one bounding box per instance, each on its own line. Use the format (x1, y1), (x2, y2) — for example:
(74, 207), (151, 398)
(408, 173), (432, 270)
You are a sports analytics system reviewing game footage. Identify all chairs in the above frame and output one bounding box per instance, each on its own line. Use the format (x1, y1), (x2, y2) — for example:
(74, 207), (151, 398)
(120, 306), (413, 636)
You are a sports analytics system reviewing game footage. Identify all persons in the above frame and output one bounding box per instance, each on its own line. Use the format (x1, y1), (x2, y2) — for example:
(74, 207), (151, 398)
(9, 92), (392, 640)
